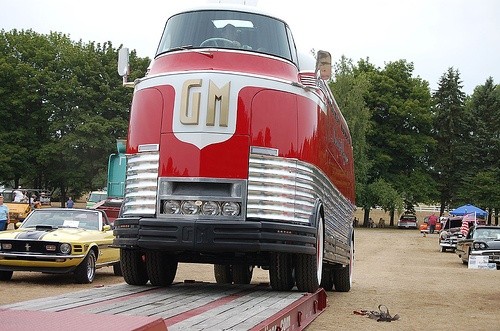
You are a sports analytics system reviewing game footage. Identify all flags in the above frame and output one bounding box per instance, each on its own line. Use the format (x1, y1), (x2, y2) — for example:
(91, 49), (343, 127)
(460, 213), (469, 236)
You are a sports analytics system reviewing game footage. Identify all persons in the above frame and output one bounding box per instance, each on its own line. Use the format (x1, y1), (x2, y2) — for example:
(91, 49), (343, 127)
(66, 197), (74, 208)
(14, 186), (39, 208)
(0, 196), (10, 231)
(354, 217), (384, 228)
(428, 213), (438, 233)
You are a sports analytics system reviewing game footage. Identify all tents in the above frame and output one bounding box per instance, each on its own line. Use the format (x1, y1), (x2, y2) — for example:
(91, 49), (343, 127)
(449, 204), (488, 230)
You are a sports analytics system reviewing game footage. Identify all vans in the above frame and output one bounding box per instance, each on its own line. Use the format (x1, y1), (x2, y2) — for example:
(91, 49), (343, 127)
(108, 4), (356, 293)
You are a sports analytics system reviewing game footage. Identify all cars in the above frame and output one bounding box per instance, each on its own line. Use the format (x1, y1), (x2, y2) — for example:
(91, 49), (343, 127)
(397, 215), (419, 229)
(0, 187), (123, 283)
(419, 216), (500, 264)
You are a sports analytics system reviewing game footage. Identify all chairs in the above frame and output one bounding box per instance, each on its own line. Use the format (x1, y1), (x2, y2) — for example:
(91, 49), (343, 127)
(79, 220), (104, 229)
(367, 220), (375, 228)
(377, 220), (386, 228)
(353, 218), (360, 228)
(47, 217), (64, 225)
